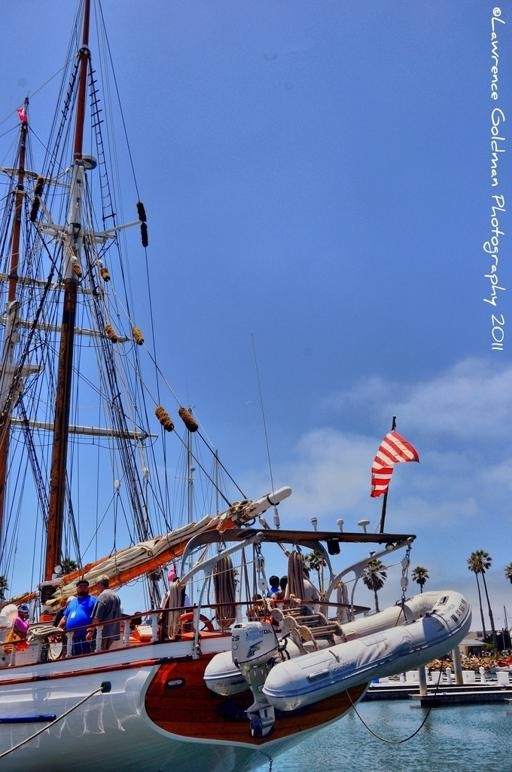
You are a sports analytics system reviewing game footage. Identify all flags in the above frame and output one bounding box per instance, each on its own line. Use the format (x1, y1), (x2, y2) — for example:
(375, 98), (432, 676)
(371, 425), (420, 496)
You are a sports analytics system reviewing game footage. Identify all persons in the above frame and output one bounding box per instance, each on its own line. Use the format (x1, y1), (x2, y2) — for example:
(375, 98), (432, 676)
(0, 574), (190, 667)
(469, 646), (511, 657)
(268, 567), (318, 615)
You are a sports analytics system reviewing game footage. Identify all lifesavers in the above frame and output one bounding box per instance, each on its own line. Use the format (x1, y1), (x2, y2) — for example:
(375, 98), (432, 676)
(41, 633), (68, 661)
(177, 612), (215, 640)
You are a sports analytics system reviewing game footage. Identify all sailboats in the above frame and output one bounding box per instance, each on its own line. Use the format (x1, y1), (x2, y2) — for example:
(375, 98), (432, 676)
(1, 0), (473, 772)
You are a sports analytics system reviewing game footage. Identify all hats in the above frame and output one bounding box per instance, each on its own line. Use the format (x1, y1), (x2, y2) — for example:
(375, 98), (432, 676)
(18, 604), (28, 613)
(94, 576), (109, 585)
